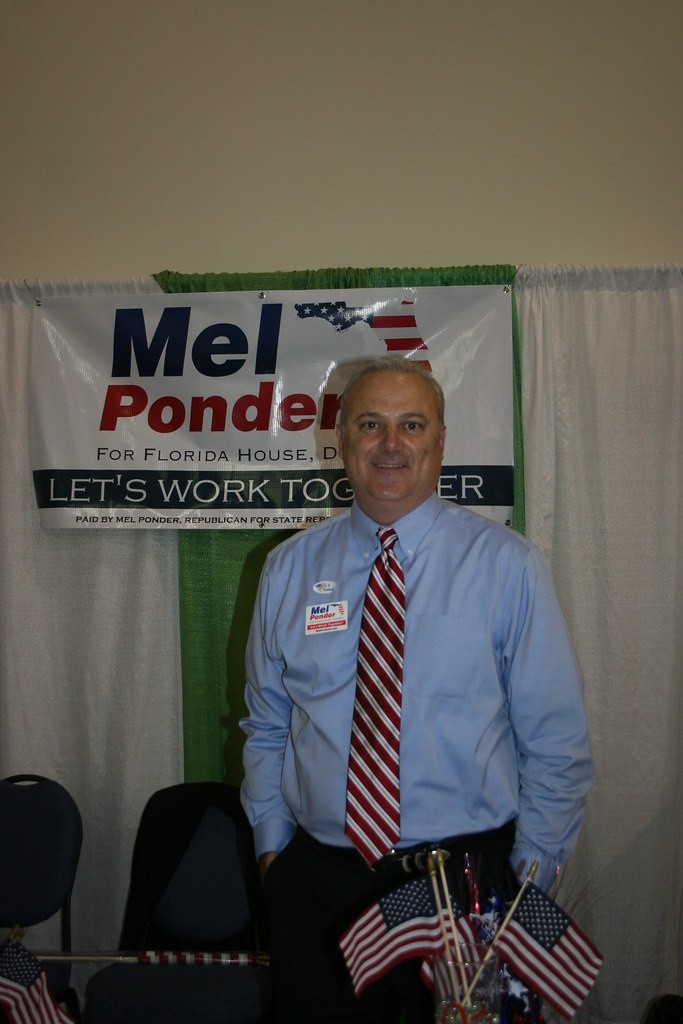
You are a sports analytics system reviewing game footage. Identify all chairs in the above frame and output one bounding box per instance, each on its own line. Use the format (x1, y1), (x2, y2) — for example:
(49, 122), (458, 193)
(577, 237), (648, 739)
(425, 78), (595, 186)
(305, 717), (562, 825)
(0, 775), (81, 1024)
(83, 781), (268, 1024)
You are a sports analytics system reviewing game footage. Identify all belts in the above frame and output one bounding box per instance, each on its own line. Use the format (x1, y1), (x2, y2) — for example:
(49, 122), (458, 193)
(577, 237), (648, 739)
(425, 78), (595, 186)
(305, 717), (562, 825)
(294, 824), (466, 874)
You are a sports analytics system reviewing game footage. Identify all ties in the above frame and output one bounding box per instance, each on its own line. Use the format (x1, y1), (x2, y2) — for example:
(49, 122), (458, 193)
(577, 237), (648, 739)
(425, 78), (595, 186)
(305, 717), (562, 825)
(345, 526), (405, 869)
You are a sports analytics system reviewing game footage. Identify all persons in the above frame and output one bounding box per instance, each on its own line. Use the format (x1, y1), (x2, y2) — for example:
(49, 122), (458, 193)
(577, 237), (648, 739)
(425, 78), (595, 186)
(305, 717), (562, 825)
(239, 354), (592, 1023)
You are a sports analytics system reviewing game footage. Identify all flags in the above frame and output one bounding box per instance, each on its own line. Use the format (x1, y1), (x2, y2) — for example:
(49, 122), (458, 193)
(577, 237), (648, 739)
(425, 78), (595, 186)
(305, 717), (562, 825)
(0, 940), (77, 1024)
(336, 872), (607, 1023)
